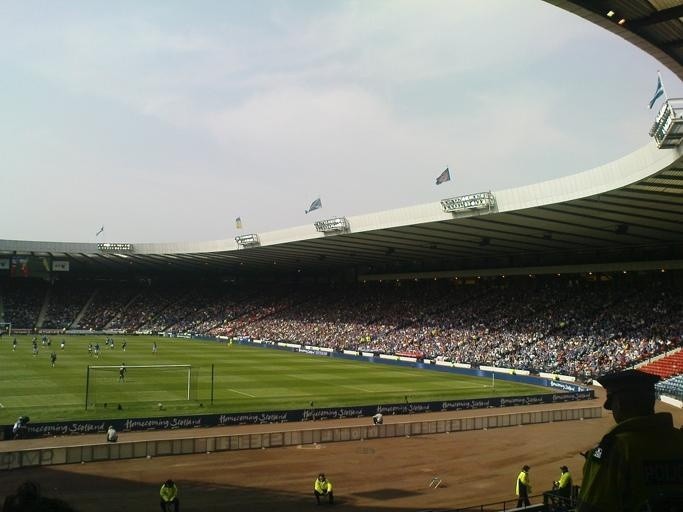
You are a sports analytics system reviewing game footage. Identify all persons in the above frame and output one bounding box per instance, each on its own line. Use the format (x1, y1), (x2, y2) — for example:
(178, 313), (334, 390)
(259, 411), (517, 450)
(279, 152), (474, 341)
(573, 368), (683, 511)
(372, 412), (383, 425)
(159, 479), (180, 512)
(515, 464), (531, 507)
(2, 480), (75, 512)
(313, 473), (334, 505)
(106, 425), (119, 442)
(119, 363), (128, 383)
(554, 465), (571, 508)
(12, 416), (29, 440)
(1, 276), (683, 378)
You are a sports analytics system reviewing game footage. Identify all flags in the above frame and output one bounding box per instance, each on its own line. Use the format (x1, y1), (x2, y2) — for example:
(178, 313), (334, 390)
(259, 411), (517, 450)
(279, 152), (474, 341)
(647, 75), (664, 110)
(233, 217), (241, 230)
(95, 226), (103, 236)
(435, 168), (451, 185)
(305, 198), (322, 215)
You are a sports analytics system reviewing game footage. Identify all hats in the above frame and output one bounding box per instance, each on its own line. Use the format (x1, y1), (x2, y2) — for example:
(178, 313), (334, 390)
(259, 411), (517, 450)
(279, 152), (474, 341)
(560, 466), (568, 472)
(596, 369), (661, 410)
(166, 479), (172, 484)
